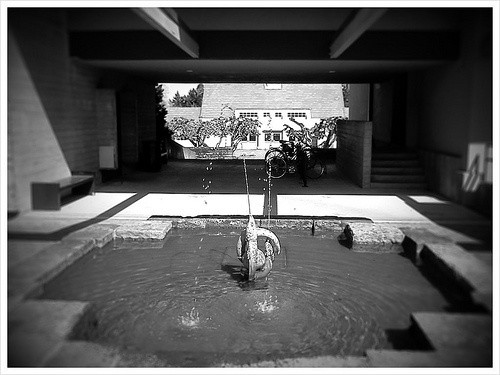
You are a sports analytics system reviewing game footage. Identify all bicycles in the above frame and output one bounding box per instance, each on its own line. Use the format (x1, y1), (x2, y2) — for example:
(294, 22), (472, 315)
(266, 134), (317, 171)
(265, 146), (324, 179)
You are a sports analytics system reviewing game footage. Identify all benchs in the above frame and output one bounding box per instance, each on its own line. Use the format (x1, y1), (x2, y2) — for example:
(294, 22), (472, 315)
(32, 170), (97, 211)
